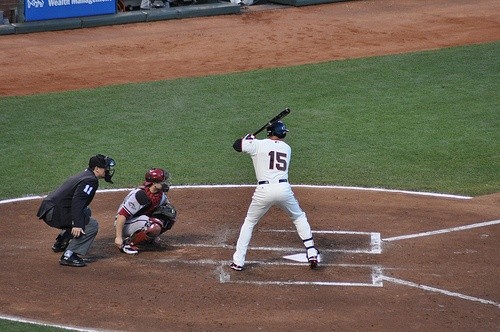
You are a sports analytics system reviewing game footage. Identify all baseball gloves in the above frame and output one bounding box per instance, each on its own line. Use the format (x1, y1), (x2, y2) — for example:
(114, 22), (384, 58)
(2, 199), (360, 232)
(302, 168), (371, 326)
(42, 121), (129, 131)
(152, 205), (177, 222)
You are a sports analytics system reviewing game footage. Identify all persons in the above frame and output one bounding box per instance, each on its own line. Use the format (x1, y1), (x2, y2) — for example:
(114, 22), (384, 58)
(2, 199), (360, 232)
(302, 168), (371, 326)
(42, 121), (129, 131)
(36, 155), (116, 267)
(114, 168), (178, 254)
(230, 121), (318, 270)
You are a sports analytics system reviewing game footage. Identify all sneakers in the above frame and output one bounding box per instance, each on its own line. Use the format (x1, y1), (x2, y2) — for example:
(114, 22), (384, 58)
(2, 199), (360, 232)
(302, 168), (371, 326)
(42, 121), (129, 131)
(230, 261), (242, 270)
(309, 258), (318, 269)
(60, 252), (85, 266)
(122, 243), (139, 254)
(52, 243), (67, 251)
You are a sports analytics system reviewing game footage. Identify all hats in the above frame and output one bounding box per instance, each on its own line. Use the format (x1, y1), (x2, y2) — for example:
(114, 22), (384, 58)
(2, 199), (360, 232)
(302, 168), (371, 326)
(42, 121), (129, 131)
(88, 154), (105, 170)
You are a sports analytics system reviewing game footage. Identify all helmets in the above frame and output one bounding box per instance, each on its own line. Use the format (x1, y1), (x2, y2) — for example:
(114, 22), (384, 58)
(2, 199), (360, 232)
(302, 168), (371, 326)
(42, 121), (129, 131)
(267, 122), (289, 139)
(144, 168), (164, 186)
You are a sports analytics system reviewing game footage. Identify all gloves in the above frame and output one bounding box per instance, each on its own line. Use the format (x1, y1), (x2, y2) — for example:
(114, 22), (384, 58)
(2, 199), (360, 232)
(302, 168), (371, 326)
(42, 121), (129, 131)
(244, 134), (255, 141)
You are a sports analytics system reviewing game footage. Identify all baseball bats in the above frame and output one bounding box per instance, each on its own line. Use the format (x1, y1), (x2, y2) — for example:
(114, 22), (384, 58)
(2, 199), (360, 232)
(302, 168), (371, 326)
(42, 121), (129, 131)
(253, 108), (291, 137)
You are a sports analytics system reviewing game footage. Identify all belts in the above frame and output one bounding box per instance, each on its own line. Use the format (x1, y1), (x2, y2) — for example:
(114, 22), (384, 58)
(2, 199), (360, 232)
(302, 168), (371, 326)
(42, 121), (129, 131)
(259, 180), (288, 185)
